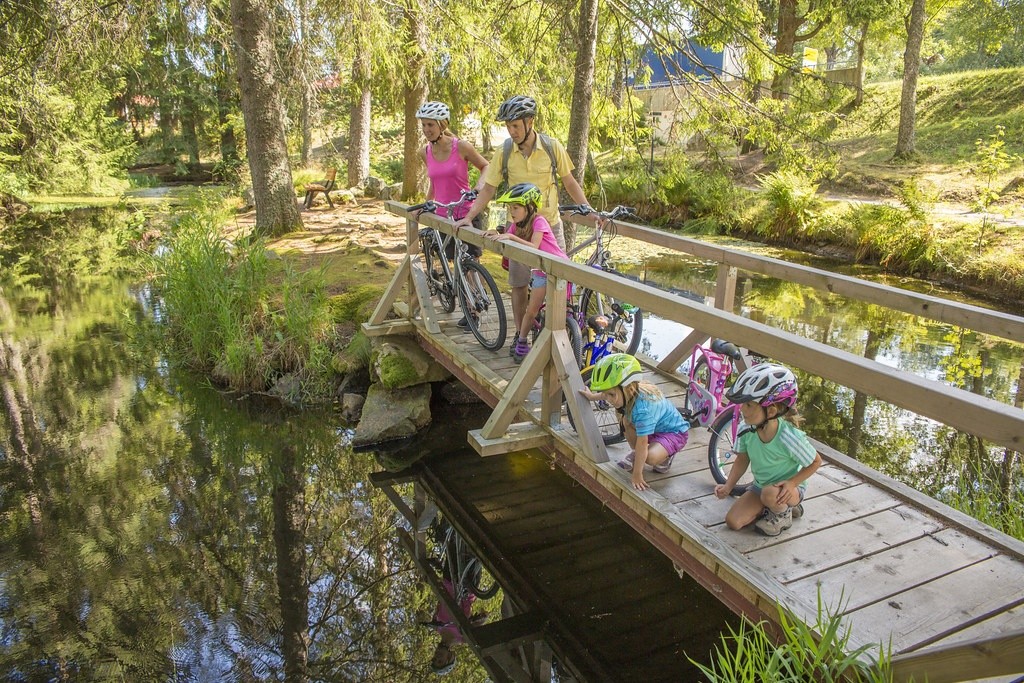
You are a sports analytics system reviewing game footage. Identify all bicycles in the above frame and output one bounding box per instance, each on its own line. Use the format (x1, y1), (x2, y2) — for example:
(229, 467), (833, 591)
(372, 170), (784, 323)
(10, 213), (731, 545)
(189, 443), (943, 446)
(684, 334), (787, 493)
(559, 202), (644, 359)
(568, 299), (644, 444)
(406, 190), (507, 351)
(525, 279), (582, 373)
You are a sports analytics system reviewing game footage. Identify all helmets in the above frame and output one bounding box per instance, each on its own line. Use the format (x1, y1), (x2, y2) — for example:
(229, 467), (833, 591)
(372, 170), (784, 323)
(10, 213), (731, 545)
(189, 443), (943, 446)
(725, 363), (798, 408)
(590, 353), (643, 390)
(416, 101), (450, 122)
(496, 182), (543, 212)
(496, 94), (537, 121)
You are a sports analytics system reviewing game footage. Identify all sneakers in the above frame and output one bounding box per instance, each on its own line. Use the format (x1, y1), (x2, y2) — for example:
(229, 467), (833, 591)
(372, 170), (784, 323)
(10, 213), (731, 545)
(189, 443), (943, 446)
(509, 336), (518, 356)
(513, 340), (528, 364)
(456, 317), (467, 327)
(463, 316), (480, 334)
(653, 455), (673, 473)
(617, 449), (635, 472)
(754, 507), (793, 535)
(758, 505), (804, 518)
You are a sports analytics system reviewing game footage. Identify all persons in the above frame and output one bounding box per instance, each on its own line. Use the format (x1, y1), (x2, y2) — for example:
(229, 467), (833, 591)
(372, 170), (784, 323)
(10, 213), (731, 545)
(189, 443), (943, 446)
(416, 101), (489, 333)
(481, 182), (571, 363)
(429, 539), (482, 674)
(577, 353), (690, 490)
(714, 362), (821, 535)
(453, 95), (606, 357)
